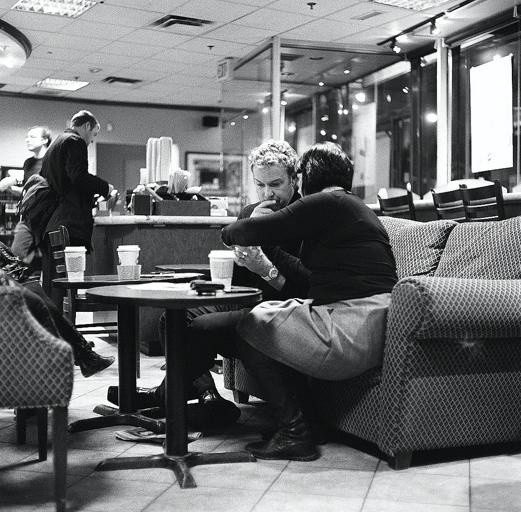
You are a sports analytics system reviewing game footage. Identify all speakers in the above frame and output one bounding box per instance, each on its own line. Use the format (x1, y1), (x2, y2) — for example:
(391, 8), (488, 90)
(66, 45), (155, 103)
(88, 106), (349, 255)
(201, 115), (220, 129)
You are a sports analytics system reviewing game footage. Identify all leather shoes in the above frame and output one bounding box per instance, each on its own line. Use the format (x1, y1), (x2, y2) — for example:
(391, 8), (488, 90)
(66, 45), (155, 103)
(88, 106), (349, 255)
(186, 395), (242, 433)
(106, 383), (160, 413)
(77, 346), (117, 377)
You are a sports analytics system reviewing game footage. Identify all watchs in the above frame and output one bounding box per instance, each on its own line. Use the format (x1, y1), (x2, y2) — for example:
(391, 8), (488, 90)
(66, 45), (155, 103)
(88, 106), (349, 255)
(261, 265), (279, 282)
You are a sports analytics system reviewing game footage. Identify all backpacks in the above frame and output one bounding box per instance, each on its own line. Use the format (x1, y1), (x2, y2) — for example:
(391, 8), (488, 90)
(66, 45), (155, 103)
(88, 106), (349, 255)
(13, 169), (57, 231)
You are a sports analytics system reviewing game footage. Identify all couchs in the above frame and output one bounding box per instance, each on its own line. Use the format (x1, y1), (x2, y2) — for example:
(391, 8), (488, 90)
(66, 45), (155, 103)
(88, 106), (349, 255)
(0, 286), (76, 506)
(222, 215), (521, 471)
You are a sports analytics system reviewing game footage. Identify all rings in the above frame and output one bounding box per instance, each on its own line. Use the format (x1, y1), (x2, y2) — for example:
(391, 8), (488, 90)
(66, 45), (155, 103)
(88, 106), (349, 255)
(242, 251), (248, 259)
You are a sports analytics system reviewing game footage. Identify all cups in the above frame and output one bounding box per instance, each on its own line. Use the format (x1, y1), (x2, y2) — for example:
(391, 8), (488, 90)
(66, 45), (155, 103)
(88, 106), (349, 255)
(63, 245), (86, 283)
(117, 244), (140, 266)
(208, 249), (236, 293)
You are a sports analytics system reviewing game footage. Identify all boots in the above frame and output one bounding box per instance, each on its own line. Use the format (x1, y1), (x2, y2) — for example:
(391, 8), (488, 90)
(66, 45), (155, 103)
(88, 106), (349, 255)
(245, 372), (322, 463)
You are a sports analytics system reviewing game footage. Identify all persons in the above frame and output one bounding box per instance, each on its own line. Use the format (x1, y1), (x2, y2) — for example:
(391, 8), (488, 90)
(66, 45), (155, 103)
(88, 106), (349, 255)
(37, 109), (116, 314)
(107, 140), (301, 424)
(219, 140), (400, 465)
(0, 241), (116, 379)
(8, 124), (52, 265)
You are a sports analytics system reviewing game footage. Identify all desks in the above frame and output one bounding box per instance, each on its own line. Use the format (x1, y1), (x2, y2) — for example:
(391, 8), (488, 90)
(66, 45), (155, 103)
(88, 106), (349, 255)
(85, 281), (262, 489)
(50, 272), (204, 434)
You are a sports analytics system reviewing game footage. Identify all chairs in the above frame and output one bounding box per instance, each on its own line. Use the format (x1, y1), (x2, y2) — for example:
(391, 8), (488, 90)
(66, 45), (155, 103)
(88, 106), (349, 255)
(375, 190), (417, 221)
(429, 186), (466, 223)
(459, 180), (505, 221)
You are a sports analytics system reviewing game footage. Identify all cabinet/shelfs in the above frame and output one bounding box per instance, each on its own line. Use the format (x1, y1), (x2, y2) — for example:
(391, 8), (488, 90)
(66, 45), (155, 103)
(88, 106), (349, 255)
(86, 213), (238, 359)
